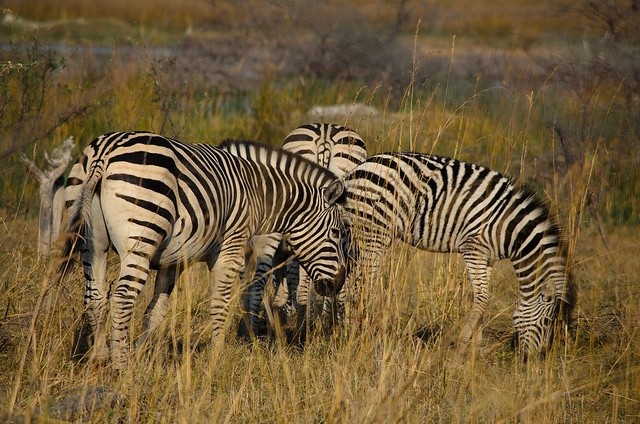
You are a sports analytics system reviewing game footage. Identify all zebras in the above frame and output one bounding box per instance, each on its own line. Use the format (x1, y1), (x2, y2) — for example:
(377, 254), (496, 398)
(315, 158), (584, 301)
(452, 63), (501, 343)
(244, 123), (368, 347)
(53, 129), (350, 382)
(308, 150), (579, 368)
